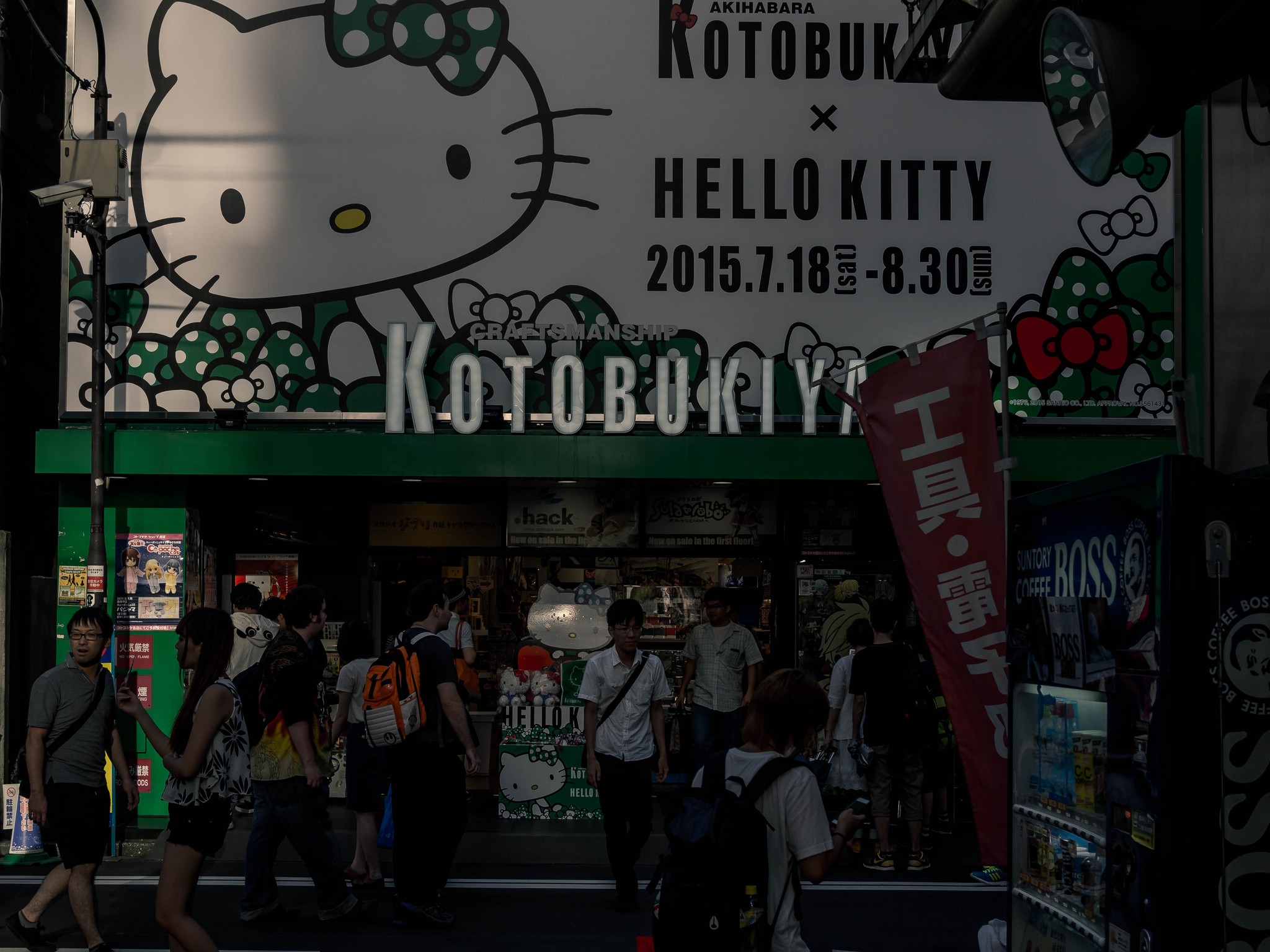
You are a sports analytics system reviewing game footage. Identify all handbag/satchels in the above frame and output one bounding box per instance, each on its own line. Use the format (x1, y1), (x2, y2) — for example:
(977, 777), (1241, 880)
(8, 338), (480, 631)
(19, 762), (47, 795)
(581, 743), (588, 768)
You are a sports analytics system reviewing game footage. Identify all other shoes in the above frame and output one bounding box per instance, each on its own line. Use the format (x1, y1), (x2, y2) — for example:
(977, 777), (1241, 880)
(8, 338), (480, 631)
(6, 914), (57, 952)
(266, 904), (301, 917)
(346, 896), (377, 914)
(342, 866), (385, 891)
(616, 891), (640, 910)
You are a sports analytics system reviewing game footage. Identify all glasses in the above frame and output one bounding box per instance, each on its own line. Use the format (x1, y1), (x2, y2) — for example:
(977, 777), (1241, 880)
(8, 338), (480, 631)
(612, 625), (644, 633)
(704, 604), (724, 610)
(68, 631), (103, 640)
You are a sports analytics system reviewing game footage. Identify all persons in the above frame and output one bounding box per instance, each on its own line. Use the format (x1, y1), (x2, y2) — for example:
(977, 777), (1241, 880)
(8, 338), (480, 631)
(6, 607), (140, 952)
(235, 595), (327, 814)
(678, 587), (762, 804)
(967, 866), (1009, 884)
(436, 581), (481, 801)
(238, 584), (358, 923)
(577, 600), (670, 912)
(116, 607), (253, 952)
(898, 629), (955, 834)
(731, 604), (763, 690)
(219, 583), (279, 829)
(330, 618), (386, 889)
(647, 668), (873, 952)
(822, 618), (873, 853)
(847, 598), (933, 870)
(363, 580), (482, 925)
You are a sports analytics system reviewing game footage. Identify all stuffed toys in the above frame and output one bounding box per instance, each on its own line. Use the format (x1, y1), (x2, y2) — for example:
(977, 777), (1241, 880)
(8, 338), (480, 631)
(531, 670), (561, 706)
(498, 667), (529, 705)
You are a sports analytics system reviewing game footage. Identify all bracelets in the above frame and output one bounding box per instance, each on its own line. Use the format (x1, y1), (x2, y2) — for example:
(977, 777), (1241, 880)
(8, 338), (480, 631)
(825, 728), (834, 733)
(830, 832), (847, 848)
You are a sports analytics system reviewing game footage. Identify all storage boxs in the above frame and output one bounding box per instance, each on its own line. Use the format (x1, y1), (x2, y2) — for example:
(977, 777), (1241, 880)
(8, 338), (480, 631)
(640, 614), (676, 639)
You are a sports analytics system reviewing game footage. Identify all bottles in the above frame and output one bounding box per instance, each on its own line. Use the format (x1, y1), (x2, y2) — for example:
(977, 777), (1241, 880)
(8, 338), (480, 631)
(1023, 817), (1108, 916)
(1123, 732), (1157, 857)
(740, 883), (766, 950)
(1030, 701), (1079, 807)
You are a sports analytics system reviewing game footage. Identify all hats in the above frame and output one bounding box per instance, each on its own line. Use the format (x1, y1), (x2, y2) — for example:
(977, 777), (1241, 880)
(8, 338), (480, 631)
(442, 580), (470, 606)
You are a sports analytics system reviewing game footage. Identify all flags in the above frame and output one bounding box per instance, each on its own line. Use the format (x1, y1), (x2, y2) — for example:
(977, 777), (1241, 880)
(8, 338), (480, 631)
(850, 335), (1008, 866)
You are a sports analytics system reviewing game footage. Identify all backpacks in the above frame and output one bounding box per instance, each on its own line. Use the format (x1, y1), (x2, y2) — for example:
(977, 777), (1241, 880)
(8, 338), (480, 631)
(653, 746), (808, 952)
(362, 630), (438, 748)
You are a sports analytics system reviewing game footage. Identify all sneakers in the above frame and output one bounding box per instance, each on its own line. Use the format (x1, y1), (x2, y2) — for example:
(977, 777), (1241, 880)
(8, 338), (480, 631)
(907, 851), (930, 870)
(393, 894), (454, 924)
(970, 866), (1007, 887)
(899, 833), (933, 849)
(875, 843), (897, 854)
(864, 850), (895, 870)
(849, 843), (861, 852)
(931, 821), (951, 834)
